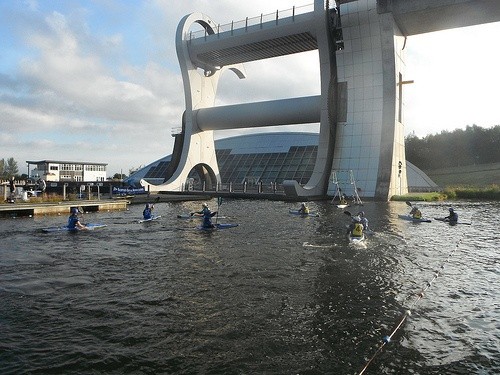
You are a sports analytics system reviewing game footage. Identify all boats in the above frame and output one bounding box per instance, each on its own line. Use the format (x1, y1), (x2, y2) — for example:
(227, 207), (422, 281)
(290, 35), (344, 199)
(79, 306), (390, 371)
(178, 223), (239, 231)
(138, 215), (161, 223)
(336, 202), (349, 209)
(349, 215), (363, 236)
(398, 215), (432, 223)
(434, 217), (470, 225)
(177, 214), (226, 219)
(289, 210), (319, 217)
(40, 224), (108, 233)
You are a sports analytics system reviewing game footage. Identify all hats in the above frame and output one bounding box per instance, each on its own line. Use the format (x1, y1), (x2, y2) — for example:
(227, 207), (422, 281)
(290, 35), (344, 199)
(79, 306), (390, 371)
(72, 208), (78, 213)
(449, 208), (453, 212)
(355, 216), (360, 222)
(414, 206), (418, 210)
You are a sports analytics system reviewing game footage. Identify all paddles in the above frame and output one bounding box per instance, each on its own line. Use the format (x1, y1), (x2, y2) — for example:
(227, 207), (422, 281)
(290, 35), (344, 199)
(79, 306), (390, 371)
(140, 197), (160, 219)
(215, 196), (223, 223)
(344, 210), (351, 217)
(406, 200), (414, 208)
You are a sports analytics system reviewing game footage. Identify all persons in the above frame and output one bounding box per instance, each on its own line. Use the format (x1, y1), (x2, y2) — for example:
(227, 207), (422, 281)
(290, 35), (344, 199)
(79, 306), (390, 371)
(7, 176), (16, 199)
(199, 203), (209, 215)
(409, 206), (422, 219)
(36, 178), (46, 193)
(446, 208), (458, 222)
(68, 208), (84, 231)
(143, 203), (152, 218)
(349, 216), (363, 236)
(204, 209), (217, 227)
(339, 195), (348, 205)
(299, 203), (309, 214)
